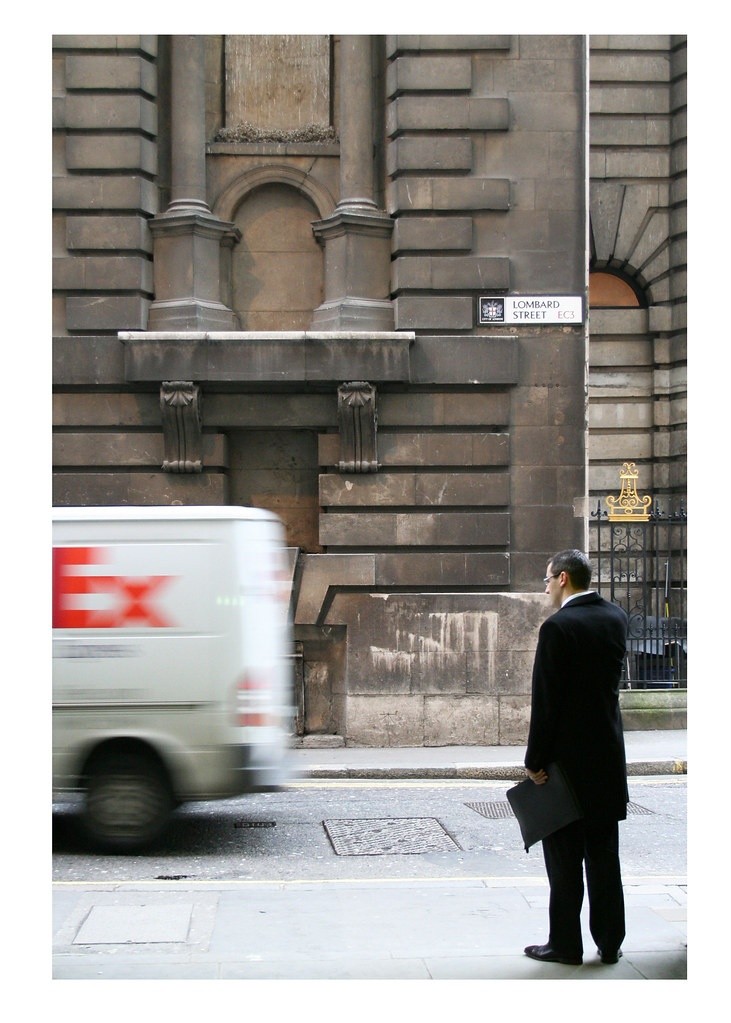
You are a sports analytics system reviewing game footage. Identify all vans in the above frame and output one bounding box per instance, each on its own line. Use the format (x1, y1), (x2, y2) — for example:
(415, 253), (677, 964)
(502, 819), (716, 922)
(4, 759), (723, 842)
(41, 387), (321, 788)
(52, 505), (291, 856)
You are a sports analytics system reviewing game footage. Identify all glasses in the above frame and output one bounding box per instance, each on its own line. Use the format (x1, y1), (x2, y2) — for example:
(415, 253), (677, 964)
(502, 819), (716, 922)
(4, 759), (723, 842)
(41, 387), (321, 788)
(543, 570), (563, 585)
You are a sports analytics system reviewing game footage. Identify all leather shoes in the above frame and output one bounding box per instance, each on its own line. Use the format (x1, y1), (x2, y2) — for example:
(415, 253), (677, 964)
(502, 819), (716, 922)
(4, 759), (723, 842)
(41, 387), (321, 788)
(524, 942), (583, 964)
(598, 948), (622, 964)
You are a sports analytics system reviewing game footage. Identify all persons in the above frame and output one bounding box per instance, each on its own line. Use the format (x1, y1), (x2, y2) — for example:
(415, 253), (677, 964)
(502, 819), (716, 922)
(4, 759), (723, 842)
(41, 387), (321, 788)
(524, 548), (628, 967)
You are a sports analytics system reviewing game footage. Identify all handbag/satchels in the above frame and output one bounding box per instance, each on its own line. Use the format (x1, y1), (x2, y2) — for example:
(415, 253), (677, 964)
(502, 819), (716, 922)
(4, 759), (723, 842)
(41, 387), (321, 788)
(507, 762), (584, 853)
